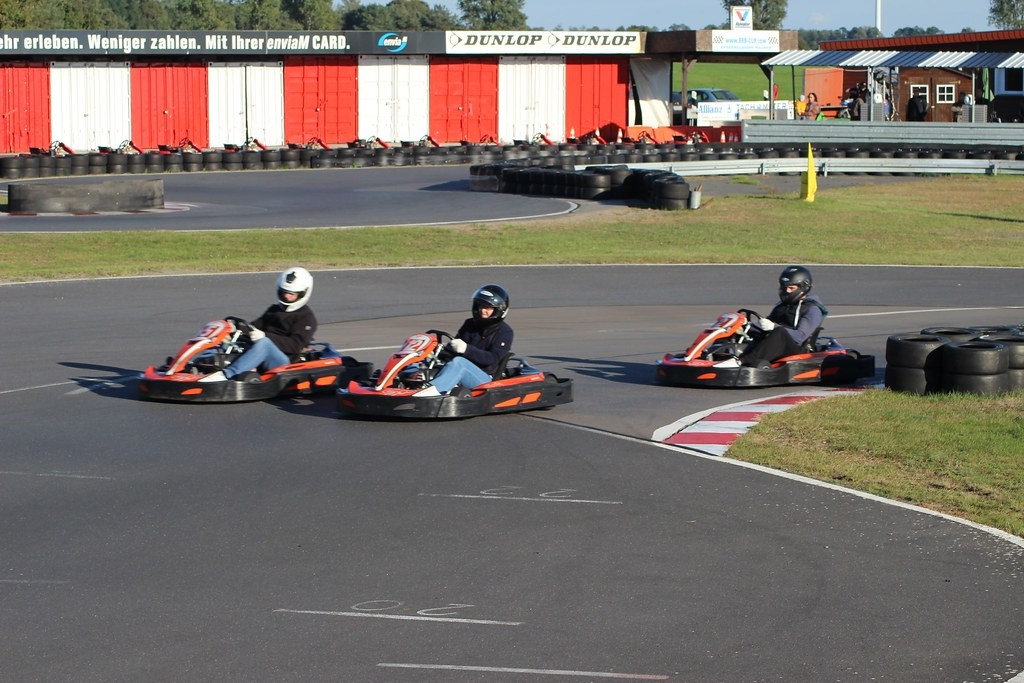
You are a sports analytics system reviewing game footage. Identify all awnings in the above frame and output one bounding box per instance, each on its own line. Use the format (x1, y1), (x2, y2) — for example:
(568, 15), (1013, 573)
(760, 48), (1024, 125)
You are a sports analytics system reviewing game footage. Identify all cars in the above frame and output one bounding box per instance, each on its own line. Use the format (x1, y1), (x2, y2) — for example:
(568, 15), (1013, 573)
(672, 88), (741, 108)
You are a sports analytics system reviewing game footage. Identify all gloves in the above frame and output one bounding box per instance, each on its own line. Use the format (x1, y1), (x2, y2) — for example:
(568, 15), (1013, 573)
(249, 326), (266, 341)
(450, 339), (467, 353)
(759, 317), (775, 330)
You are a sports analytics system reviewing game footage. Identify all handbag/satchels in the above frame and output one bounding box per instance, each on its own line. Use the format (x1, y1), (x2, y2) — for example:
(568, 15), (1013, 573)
(816, 112), (823, 121)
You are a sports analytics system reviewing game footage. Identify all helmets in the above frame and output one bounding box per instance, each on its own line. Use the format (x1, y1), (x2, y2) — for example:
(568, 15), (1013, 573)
(780, 266), (811, 305)
(472, 284), (510, 321)
(276, 267), (313, 312)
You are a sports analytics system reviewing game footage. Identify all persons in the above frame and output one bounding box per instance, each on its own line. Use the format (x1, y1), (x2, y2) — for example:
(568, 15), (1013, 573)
(797, 93), (822, 120)
(156, 267), (318, 383)
(845, 82), (892, 121)
(712, 266), (828, 368)
(906, 88), (928, 122)
(399, 284), (513, 397)
(951, 92), (965, 122)
(975, 87), (993, 122)
